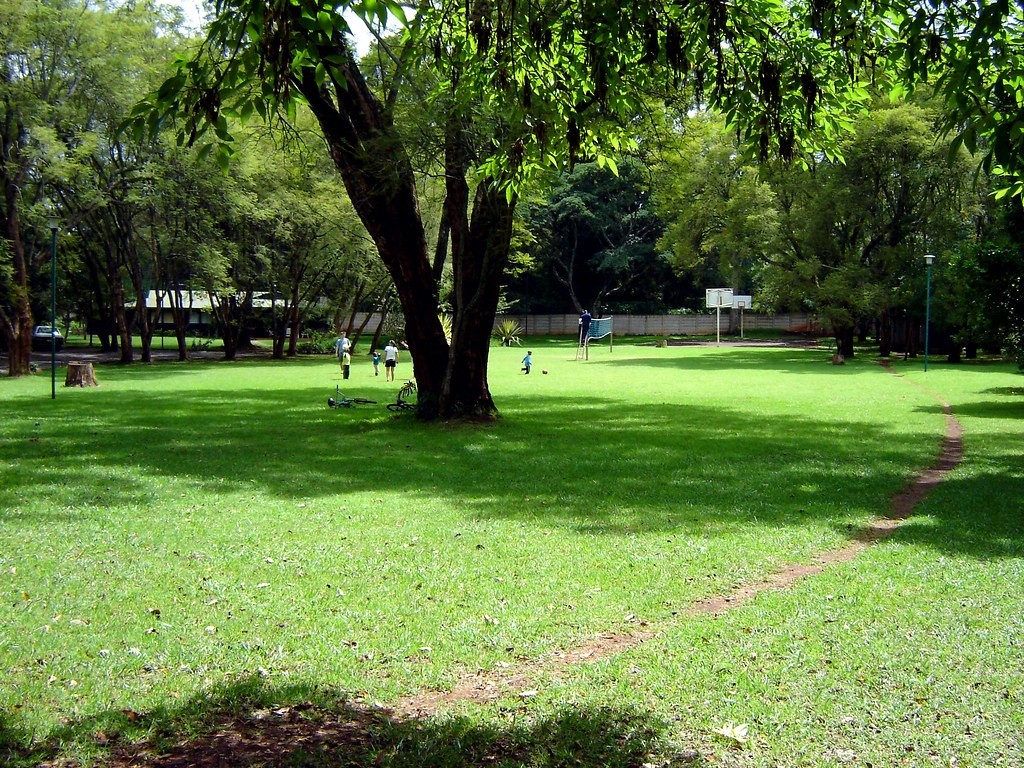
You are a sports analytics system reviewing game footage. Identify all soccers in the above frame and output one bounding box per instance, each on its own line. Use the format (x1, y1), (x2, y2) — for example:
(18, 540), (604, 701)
(542, 369), (548, 375)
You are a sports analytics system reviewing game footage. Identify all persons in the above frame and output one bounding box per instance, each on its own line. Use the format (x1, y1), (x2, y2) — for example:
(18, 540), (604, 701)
(579, 308), (592, 342)
(521, 350), (532, 374)
(336, 331), (399, 383)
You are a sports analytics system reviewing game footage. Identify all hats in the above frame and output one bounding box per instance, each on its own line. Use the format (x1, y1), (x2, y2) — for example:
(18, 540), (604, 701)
(340, 331), (346, 334)
(528, 351), (532, 353)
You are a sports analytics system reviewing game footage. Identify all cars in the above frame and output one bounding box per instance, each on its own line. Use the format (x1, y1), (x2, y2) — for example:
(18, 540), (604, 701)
(34, 325), (64, 340)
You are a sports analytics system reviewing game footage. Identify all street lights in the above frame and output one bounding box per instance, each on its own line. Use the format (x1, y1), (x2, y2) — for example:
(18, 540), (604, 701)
(923, 253), (936, 372)
(45, 215), (61, 399)
